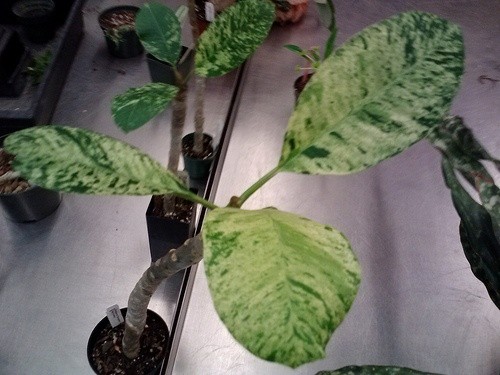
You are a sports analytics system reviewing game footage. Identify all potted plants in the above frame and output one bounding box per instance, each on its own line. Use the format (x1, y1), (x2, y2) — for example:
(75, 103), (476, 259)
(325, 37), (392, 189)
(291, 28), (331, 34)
(182, 0), (215, 179)
(3, 10), (465, 375)
(111, 0), (278, 263)
(283, 0), (338, 111)
